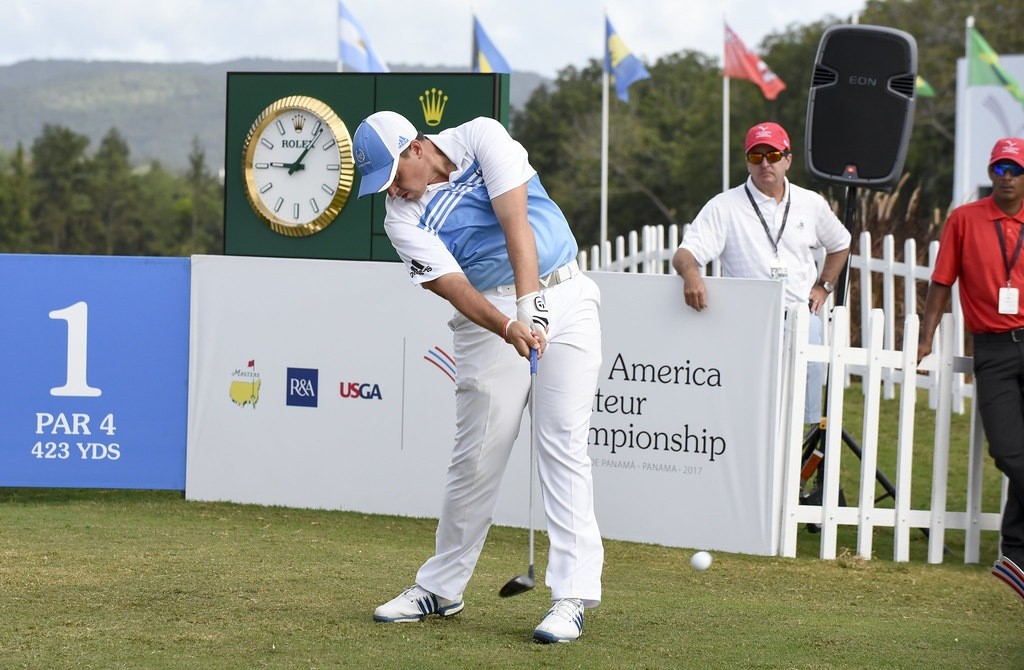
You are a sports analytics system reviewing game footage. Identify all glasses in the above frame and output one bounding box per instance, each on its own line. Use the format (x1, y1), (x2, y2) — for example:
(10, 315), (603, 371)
(989, 163), (1024, 176)
(746, 150), (790, 165)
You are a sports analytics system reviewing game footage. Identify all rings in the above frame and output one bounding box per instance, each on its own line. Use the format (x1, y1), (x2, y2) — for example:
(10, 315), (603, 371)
(813, 300), (818, 303)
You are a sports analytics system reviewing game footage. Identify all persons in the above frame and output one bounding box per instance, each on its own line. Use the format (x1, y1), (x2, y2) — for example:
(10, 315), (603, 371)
(672, 122), (852, 424)
(352, 111), (604, 643)
(917, 138), (1024, 573)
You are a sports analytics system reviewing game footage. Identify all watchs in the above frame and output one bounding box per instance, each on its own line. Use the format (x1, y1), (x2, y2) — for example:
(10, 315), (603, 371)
(814, 280), (834, 294)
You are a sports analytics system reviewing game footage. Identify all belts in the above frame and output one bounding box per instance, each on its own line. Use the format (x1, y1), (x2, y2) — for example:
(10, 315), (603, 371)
(972, 329), (1024, 344)
(475, 259), (581, 299)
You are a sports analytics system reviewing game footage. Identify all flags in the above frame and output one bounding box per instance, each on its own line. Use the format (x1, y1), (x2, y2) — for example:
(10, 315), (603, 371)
(603, 18), (651, 103)
(337, 2), (391, 73)
(968, 28), (1024, 112)
(724, 23), (786, 101)
(472, 15), (512, 73)
(916, 75), (936, 98)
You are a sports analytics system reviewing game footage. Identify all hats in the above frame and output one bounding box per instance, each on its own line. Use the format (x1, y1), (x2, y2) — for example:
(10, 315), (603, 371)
(988, 137), (1024, 169)
(350, 111), (418, 200)
(745, 122), (791, 154)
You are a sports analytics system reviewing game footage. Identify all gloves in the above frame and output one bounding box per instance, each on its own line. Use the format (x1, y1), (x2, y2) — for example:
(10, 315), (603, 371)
(515, 292), (549, 344)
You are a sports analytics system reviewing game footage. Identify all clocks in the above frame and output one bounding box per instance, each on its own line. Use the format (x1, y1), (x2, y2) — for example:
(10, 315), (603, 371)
(242, 96), (355, 238)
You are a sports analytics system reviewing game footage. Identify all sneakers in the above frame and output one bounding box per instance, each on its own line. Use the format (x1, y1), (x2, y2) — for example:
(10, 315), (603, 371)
(532, 596), (585, 643)
(373, 583), (465, 623)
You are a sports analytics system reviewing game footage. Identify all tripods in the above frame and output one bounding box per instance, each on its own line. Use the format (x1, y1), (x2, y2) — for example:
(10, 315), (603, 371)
(800, 186), (935, 546)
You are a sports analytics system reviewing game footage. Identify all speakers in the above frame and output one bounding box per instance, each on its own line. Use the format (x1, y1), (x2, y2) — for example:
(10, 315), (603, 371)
(804, 24), (918, 194)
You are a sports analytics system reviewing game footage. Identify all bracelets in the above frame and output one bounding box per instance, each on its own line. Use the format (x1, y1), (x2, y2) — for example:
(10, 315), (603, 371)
(503, 319), (517, 343)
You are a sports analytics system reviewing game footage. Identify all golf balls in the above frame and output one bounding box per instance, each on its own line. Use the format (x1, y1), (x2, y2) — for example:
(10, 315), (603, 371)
(690, 551), (711, 570)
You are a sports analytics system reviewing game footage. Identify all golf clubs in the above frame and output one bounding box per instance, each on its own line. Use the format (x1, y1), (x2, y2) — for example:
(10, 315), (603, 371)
(499, 346), (538, 598)
